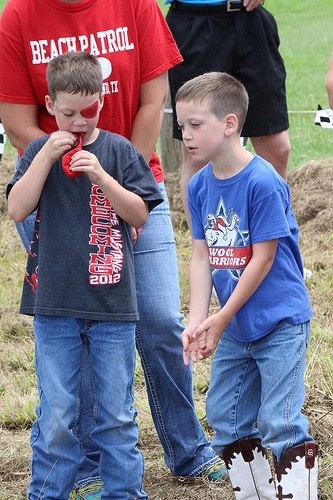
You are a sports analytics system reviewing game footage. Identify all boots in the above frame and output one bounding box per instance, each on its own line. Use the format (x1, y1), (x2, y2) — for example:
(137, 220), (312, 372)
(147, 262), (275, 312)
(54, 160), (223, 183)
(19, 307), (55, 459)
(273, 441), (319, 500)
(222, 436), (277, 500)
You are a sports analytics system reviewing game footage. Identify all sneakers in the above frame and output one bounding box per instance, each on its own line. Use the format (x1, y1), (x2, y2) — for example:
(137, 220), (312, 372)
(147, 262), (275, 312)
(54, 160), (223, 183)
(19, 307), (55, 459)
(198, 458), (230, 482)
(76, 480), (105, 500)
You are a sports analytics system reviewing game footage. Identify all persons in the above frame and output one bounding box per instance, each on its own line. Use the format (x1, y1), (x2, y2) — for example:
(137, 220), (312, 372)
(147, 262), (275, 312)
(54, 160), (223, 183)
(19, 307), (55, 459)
(175, 71), (319, 500)
(0, 0), (230, 500)
(164, 0), (312, 280)
(6, 51), (164, 500)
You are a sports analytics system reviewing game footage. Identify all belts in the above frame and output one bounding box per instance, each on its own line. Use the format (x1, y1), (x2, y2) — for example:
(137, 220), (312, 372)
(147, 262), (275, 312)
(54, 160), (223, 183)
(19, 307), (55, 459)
(171, 0), (245, 12)
